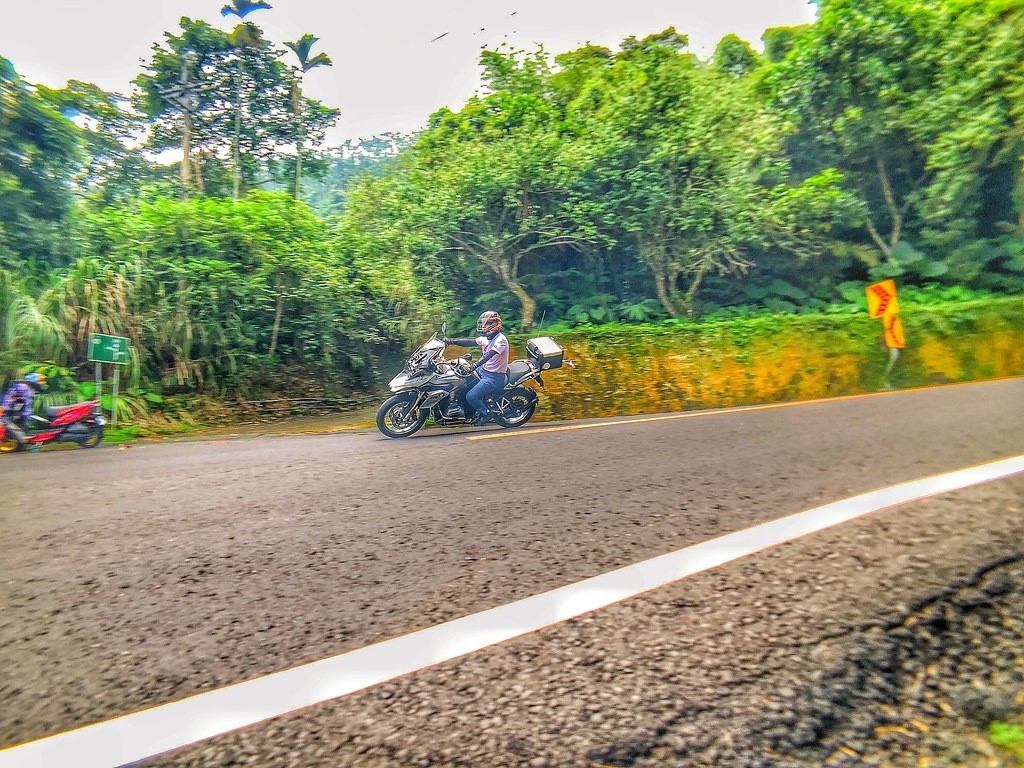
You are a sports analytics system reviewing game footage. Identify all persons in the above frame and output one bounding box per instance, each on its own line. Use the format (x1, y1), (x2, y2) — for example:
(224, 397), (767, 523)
(0, 373), (49, 451)
(442, 310), (509, 425)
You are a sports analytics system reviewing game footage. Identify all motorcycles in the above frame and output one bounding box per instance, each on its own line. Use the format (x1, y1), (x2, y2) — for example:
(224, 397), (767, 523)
(376, 322), (565, 438)
(0, 374), (107, 452)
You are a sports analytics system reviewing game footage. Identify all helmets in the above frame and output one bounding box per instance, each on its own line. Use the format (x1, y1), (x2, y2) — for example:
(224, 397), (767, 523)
(25, 373), (49, 391)
(476, 310), (504, 340)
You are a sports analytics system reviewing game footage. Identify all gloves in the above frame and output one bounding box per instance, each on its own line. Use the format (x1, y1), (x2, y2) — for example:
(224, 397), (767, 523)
(460, 363), (476, 375)
(442, 338), (454, 347)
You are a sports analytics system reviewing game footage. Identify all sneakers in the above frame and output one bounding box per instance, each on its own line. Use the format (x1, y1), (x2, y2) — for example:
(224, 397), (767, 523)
(474, 412), (498, 426)
